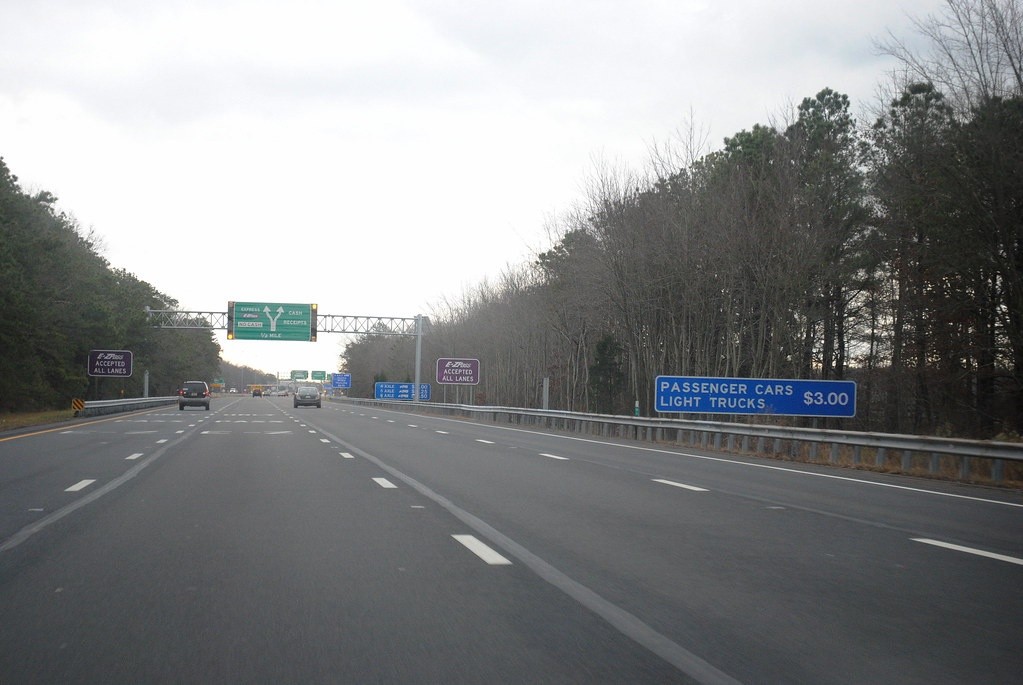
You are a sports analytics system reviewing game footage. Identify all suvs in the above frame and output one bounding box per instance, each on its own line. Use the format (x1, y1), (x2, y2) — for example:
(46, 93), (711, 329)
(178, 380), (209, 411)
(252, 388), (262, 398)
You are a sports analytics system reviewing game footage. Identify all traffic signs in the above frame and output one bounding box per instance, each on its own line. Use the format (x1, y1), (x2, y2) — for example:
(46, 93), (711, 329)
(233, 302), (310, 341)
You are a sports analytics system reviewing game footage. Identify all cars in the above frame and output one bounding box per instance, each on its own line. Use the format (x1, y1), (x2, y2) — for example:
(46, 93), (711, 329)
(229, 388), (238, 393)
(293, 387), (321, 408)
(277, 390), (288, 397)
(262, 391), (270, 396)
